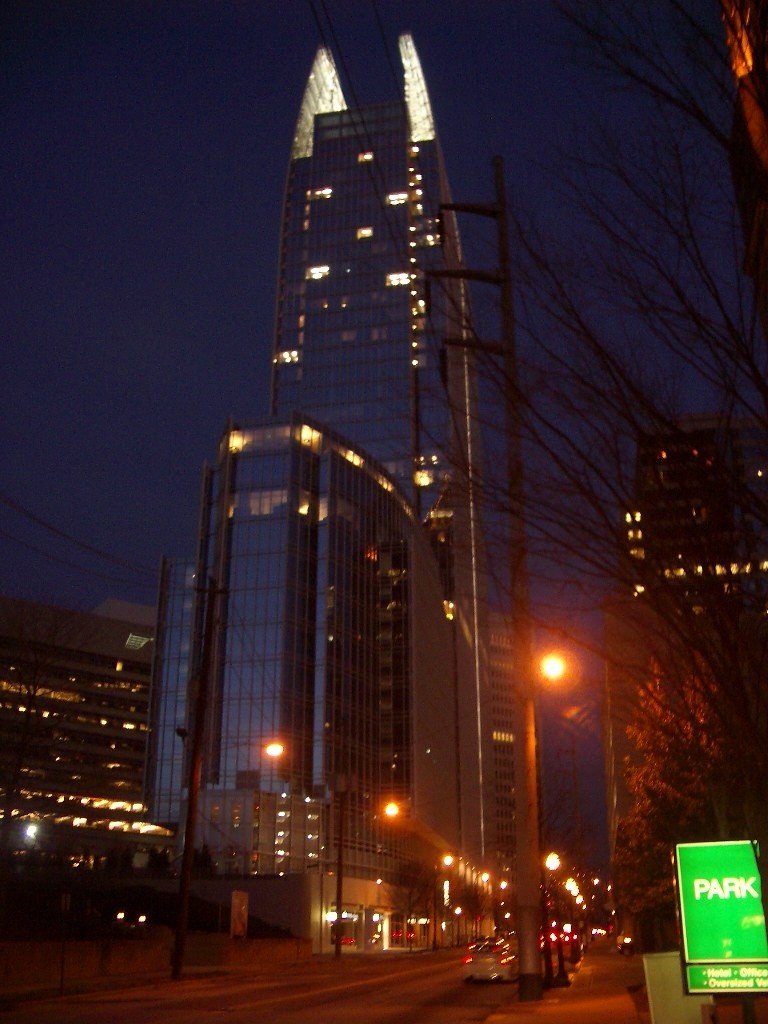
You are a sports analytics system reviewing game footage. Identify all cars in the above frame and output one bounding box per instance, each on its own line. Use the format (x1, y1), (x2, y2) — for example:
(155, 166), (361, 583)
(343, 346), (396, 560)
(460, 935), (518, 985)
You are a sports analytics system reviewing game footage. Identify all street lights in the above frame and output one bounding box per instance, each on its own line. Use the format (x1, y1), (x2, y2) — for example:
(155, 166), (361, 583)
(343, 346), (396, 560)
(332, 790), (401, 960)
(532, 649), (569, 985)
(433, 854), (455, 949)
(169, 739), (284, 981)
